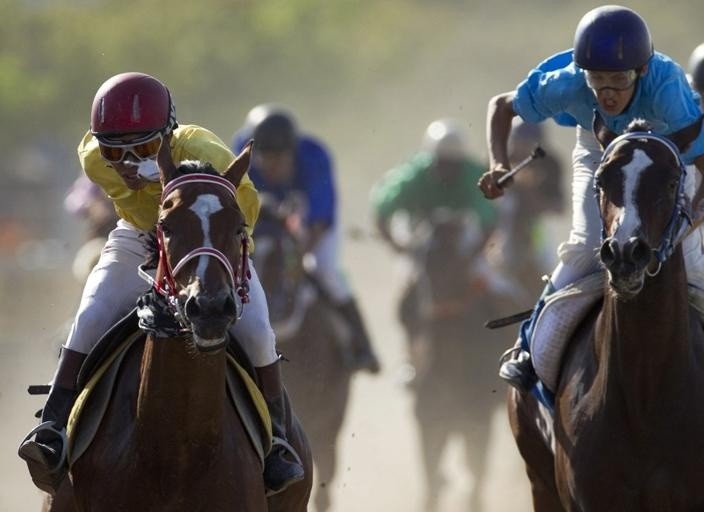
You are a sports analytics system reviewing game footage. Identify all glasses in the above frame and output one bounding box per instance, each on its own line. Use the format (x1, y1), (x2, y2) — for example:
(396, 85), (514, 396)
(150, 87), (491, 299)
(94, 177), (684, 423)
(582, 69), (639, 90)
(99, 132), (163, 163)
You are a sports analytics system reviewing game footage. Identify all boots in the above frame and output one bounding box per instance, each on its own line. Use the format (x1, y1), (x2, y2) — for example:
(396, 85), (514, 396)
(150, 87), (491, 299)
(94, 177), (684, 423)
(19, 344), (88, 494)
(498, 274), (557, 397)
(248, 350), (305, 491)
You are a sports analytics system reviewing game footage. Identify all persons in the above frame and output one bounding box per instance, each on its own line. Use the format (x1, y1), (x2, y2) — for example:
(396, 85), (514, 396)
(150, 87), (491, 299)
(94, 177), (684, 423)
(228, 103), (380, 373)
(479, 5), (704, 393)
(370, 118), (498, 389)
(16, 72), (304, 497)
(495, 118), (566, 295)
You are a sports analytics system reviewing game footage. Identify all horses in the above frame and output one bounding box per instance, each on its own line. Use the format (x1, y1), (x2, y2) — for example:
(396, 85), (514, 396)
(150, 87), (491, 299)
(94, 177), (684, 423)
(396, 209), (530, 512)
(221, 189), (362, 511)
(36, 131), (317, 512)
(503, 106), (703, 512)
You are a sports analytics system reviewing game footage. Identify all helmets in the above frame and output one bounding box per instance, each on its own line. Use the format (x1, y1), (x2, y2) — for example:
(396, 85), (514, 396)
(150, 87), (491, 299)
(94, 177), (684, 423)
(90, 72), (179, 137)
(573, 5), (654, 71)
